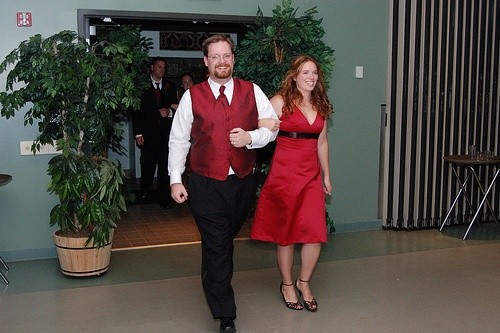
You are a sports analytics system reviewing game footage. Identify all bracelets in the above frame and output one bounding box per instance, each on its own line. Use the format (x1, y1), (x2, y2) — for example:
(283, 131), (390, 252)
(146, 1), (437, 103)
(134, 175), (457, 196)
(248, 135), (252, 146)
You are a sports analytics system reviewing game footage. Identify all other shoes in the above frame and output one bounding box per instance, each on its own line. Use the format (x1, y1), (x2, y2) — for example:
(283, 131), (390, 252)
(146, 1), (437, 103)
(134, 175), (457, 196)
(218, 320), (236, 333)
(141, 190), (151, 199)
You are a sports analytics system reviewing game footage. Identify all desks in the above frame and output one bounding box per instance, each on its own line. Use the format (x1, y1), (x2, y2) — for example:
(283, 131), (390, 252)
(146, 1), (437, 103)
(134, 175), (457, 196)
(439, 155), (500, 240)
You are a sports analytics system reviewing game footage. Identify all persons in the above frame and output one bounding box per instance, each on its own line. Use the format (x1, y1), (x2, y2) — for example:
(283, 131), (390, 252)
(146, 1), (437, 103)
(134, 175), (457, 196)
(257, 55), (335, 312)
(132, 56), (198, 210)
(165, 34), (285, 333)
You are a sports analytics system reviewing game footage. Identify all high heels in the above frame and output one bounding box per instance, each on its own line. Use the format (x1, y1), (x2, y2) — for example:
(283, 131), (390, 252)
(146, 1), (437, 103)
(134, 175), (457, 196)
(280, 282), (303, 310)
(295, 278), (318, 312)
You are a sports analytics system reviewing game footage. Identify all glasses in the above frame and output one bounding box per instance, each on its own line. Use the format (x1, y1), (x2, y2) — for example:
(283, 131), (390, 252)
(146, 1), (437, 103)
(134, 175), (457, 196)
(206, 54), (233, 61)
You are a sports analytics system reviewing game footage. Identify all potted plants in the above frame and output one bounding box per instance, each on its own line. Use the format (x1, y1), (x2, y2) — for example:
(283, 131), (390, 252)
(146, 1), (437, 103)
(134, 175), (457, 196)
(0, 26), (155, 277)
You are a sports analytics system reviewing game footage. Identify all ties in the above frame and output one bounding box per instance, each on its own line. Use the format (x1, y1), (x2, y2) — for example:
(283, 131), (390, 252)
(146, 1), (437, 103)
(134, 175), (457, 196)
(216, 85), (229, 110)
(155, 82), (160, 102)
